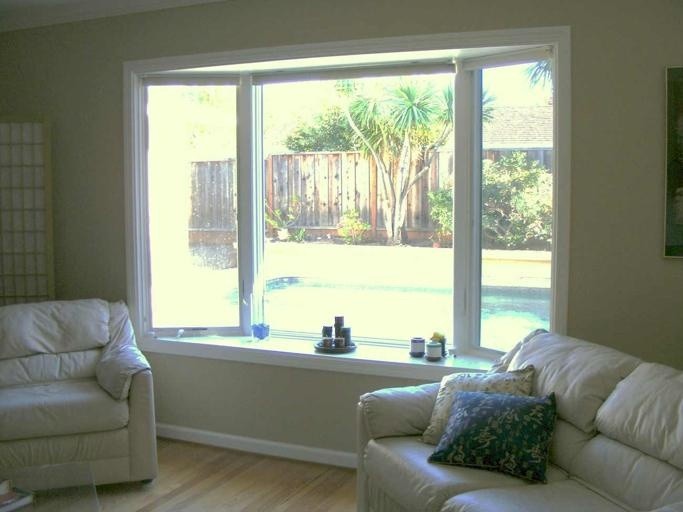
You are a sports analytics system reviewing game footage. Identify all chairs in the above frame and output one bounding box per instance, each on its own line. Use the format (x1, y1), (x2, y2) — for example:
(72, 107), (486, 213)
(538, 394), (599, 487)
(1, 298), (158, 491)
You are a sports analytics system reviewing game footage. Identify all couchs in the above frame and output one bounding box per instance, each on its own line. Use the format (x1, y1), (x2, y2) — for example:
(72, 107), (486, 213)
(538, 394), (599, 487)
(356, 329), (683, 512)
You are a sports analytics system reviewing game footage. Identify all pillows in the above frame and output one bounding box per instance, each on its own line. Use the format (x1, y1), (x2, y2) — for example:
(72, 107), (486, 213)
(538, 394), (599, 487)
(417, 365), (557, 484)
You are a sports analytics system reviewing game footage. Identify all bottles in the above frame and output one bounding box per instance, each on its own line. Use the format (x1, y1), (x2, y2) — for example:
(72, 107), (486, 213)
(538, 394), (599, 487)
(320, 316), (353, 350)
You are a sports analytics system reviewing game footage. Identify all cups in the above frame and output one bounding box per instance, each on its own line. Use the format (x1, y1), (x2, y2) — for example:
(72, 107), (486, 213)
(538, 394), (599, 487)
(409, 337), (425, 356)
(425, 341), (444, 360)
